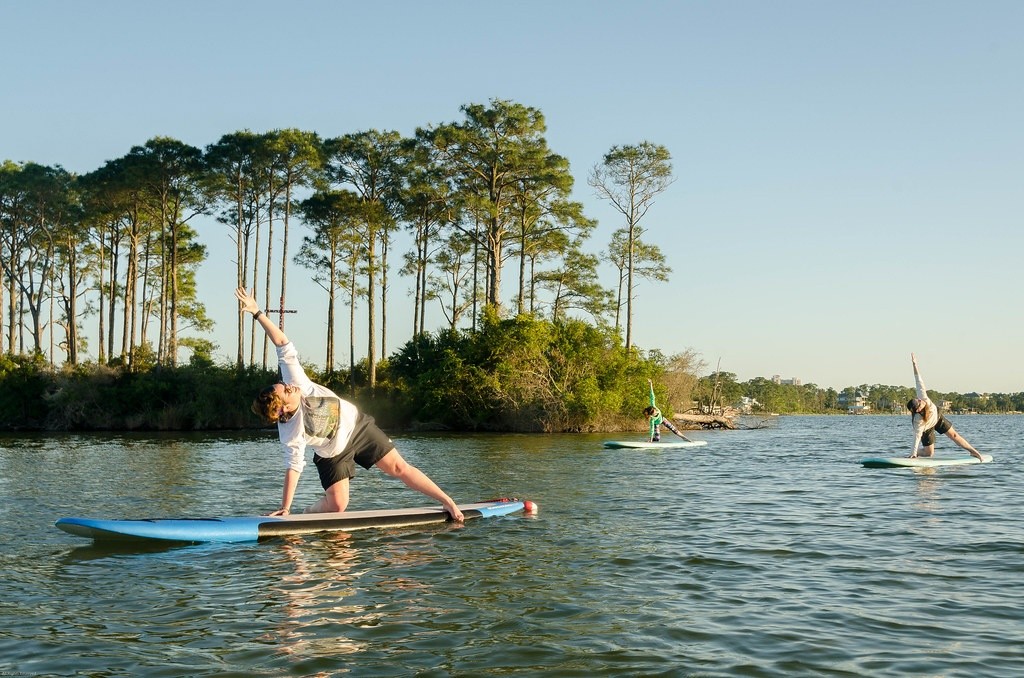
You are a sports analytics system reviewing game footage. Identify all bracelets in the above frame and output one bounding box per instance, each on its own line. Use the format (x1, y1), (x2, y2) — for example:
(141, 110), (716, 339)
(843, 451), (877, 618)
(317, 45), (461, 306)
(252, 309), (262, 320)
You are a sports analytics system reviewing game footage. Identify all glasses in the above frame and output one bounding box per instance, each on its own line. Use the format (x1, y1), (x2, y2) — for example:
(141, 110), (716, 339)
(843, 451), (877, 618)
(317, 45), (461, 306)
(278, 379), (292, 394)
(916, 397), (921, 410)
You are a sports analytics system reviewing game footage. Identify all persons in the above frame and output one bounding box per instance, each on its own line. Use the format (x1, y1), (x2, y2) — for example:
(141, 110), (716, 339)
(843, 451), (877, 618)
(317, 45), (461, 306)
(906, 352), (985, 463)
(235, 284), (465, 524)
(642, 378), (692, 442)
